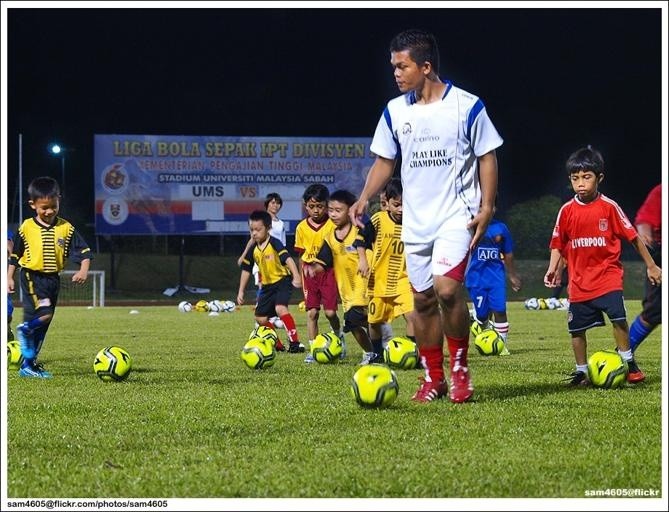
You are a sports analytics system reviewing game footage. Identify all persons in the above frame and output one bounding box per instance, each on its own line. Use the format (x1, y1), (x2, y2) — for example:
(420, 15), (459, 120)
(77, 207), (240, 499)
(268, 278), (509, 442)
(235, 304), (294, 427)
(7, 176), (96, 382)
(347, 29), (505, 405)
(543, 149), (662, 388)
(7, 229), (16, 351)
(237, 192), (287, 329)
(615, 183), (661, 352)
(237, 209), (307, 353)
(464, 186), (521, 356)
(294, 178), (417, 368)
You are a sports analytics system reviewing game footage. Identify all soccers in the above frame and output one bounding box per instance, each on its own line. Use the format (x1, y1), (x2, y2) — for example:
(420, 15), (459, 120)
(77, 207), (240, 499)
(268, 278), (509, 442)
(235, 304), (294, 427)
(249, 326), (277, 347)
(242, 340), (276, 370)
(588, 349), (628, 389)
(310, 334), (343, 364)
(475, 329), (504, 356)
(268, 315), (283, 328)
(351, 363), (400, 408)
(382, 337), (420, 370)
(471, 319), (496, 340)
(177, 299), (236, 314)
(299, 301), (308, 312)
(6, 341), (27, 371)
(524, 296), (572, 311)
(94, 346), (132, 382)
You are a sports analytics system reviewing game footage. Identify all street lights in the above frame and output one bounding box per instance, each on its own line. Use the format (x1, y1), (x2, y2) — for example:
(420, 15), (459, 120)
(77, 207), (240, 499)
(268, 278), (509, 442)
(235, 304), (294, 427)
(51, 146), (65, 217)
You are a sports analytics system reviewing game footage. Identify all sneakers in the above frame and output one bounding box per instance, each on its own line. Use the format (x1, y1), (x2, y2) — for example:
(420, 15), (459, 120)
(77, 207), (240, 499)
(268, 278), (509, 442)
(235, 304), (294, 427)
(338, 348), (347, 360)
(275, 339), (286, 352)
(558, 370), (592, 389)
(359, 347), (385, 367)
(17, 361), (54, 380)
(15, 321), (37, 359)
(303, 352), (316, 364)
(614, 346), (647, 382)
(409, 377), (449, 406)
(287, 341), (305, 353)
(449, 361), (475, 403)
(499, 346), (510, 356)
